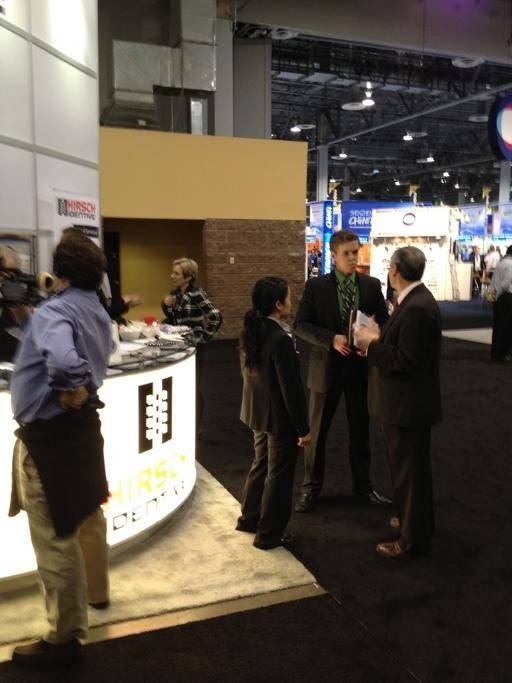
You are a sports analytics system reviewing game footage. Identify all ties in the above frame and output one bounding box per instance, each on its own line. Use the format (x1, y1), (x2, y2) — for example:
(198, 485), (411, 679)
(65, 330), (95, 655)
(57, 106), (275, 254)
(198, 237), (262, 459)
(343, 278), (353, 328)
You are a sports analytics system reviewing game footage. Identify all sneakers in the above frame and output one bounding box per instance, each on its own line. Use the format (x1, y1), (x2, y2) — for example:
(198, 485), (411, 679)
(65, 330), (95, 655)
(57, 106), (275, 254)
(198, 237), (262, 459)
(12, 600), (111, 662)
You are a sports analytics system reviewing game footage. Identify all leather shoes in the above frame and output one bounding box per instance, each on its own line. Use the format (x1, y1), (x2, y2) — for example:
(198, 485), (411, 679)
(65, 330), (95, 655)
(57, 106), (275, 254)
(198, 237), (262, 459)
(279, 485), (409, 558)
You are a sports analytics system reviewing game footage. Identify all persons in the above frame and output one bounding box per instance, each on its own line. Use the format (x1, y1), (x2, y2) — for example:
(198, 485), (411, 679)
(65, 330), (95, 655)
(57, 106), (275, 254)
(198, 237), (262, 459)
(455, 244), (503, 295)
(374, 246), (443, 561)
(307, 248), (322, 280)
(289, 228), (392, 512)
(161, 256), (223, 461)
(487, 245), (512, 365)
(234, 276), (311, 549)
(64, 227), (140, 324)
(1, 244), (43, 363)
(11, 226), (113, 668)
